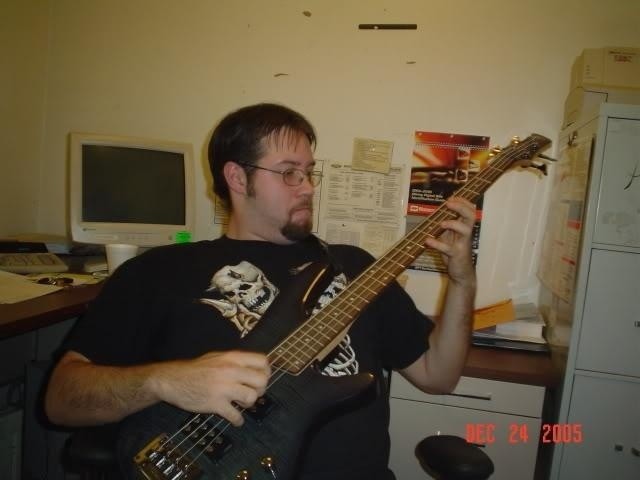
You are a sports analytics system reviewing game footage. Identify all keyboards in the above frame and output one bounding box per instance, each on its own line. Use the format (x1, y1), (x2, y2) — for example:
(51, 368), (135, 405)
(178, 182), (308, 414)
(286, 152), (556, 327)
(0, 252), (68, 274)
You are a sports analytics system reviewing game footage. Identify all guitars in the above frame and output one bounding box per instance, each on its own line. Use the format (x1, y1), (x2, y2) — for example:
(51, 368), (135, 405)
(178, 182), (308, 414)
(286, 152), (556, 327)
(134, 133), (557, 480)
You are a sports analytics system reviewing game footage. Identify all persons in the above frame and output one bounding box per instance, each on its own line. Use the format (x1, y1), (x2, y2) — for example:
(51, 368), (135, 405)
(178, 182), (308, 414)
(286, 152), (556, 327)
(43, 102), (478, 479)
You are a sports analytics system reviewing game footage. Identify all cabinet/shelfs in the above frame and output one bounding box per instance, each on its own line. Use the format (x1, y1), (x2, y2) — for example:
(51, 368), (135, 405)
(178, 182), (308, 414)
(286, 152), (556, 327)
(539, 104), (640, 480)
(389, 363), (547, 480)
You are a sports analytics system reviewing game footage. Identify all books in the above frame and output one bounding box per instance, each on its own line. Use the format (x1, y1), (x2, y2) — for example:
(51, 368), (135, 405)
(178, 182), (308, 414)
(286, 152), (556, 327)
(496, 301), (547, 339)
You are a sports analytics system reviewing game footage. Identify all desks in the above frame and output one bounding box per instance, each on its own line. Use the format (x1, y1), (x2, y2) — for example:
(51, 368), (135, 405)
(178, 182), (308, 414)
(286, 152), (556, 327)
(0, 271), (110, 480)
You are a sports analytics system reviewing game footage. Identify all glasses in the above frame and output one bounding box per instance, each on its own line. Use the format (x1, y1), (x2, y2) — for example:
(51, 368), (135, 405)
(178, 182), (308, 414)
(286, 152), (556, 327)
(244, 163), (323, 187)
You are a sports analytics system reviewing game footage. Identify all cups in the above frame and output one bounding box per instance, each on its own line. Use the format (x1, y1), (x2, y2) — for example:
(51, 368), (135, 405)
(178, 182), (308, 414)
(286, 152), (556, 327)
(106, 243), (138, 276)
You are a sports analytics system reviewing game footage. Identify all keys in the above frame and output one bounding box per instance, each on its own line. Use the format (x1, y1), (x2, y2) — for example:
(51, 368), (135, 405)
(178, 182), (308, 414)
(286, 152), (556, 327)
(28, 276), (56, 285)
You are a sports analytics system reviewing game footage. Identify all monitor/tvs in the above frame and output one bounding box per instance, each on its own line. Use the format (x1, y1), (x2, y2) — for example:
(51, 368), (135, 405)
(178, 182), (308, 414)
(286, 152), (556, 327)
(69, 131), (196, 277)
